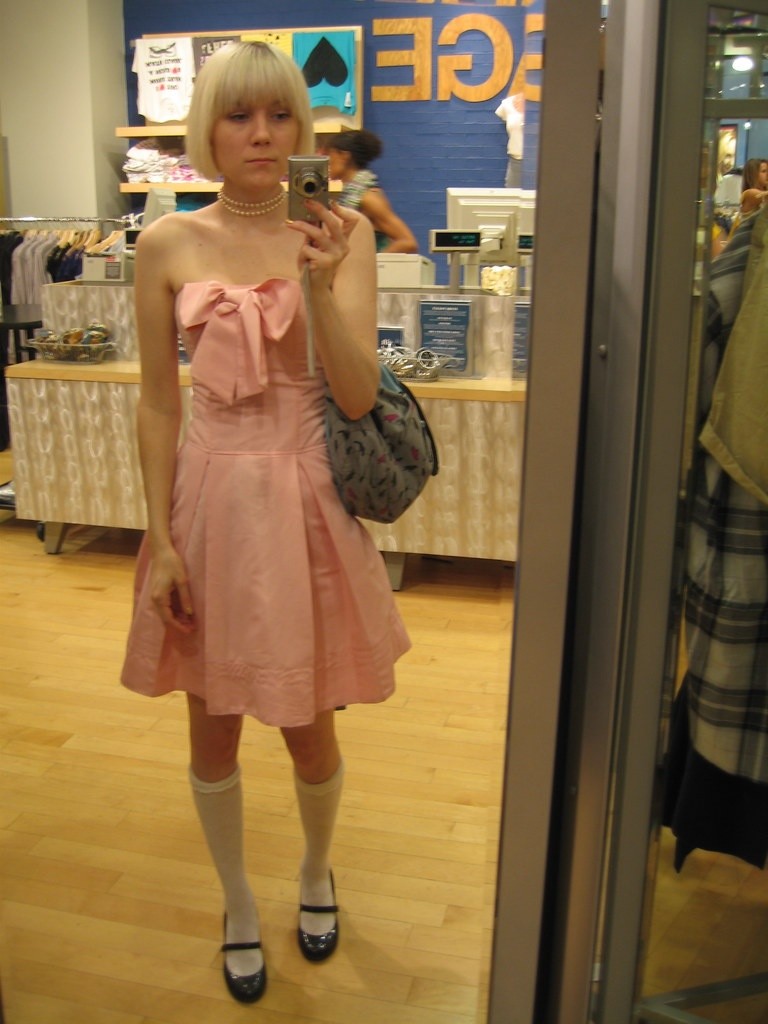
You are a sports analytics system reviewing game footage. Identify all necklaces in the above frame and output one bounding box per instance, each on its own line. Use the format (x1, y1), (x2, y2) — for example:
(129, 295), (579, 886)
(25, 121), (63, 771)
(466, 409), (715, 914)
(217, 187), (286, 216)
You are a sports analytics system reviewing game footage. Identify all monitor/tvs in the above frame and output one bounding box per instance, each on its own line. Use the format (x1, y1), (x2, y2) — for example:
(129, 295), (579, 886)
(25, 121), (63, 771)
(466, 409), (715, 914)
(141, 187), (177, 229)
(445, 187), (536, 268)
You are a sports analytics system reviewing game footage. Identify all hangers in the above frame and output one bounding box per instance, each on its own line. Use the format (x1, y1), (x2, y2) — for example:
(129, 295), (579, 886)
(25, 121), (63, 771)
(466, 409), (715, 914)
(0, 218), (130, 263)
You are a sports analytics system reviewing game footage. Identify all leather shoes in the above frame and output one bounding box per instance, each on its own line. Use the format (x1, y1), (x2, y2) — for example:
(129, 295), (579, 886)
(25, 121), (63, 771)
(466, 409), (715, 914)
(296, 867), (340, 962)
(220, 905), (267, 1005)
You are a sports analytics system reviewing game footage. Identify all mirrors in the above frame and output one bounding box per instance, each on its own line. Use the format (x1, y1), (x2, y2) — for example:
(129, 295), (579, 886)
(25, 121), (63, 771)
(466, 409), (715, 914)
(585, 4), (768, 1023)
(3, 1), (600, 1024)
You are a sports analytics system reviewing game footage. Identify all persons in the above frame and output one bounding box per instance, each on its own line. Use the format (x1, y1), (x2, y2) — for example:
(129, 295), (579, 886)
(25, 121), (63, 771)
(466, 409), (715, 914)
(120, 41), (413, 1003)
(494, 92), (525, 189)
(728, 157), (768, 241)
(714, 166), (744, 206)
(321, 130), (420, 255)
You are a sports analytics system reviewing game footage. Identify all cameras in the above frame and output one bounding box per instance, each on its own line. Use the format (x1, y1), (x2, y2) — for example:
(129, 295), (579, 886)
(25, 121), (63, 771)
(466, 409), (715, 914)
(288, 155), (329, 224)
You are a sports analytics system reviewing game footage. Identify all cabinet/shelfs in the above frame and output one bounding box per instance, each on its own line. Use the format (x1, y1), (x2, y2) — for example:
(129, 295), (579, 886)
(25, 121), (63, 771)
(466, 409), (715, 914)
(118, 119), (351, 249)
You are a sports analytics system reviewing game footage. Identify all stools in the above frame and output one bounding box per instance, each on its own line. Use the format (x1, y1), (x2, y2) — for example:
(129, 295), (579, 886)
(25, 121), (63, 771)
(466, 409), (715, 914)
(1, 302), (45, 453)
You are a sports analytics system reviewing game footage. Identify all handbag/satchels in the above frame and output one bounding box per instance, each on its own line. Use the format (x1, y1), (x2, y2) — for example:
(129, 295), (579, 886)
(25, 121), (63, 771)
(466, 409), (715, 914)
(319, 356), (444, 526)
